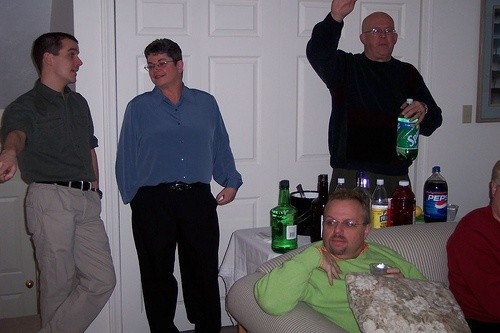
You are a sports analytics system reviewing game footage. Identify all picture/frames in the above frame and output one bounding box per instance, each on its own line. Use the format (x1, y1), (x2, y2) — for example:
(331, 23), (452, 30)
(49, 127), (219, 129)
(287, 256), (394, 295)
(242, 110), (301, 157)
(476, 0), (500, 122)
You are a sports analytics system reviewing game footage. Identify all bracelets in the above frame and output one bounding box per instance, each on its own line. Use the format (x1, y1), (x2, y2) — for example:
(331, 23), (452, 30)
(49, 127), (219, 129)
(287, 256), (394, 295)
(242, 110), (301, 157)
(424, 104), (428, 114)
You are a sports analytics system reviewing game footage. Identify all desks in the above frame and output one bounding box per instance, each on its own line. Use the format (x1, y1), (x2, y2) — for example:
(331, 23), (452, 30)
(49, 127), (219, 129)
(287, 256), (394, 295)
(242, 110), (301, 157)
(219, 226), (287, 332)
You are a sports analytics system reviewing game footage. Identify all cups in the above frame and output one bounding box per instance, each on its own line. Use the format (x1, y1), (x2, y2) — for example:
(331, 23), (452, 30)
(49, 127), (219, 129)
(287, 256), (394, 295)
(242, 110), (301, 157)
(289, 189), (320, 235)
(446, 203), (458, 221)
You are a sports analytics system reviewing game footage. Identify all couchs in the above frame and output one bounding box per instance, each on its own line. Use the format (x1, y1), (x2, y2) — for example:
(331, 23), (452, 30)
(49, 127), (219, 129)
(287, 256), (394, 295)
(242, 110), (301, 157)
(224, 219), (457, 333)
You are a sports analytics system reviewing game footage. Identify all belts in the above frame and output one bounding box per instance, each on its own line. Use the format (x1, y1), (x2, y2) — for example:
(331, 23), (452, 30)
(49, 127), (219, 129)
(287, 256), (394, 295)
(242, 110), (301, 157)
(36, 180), (93, 191)
(152, 184), (204, 192)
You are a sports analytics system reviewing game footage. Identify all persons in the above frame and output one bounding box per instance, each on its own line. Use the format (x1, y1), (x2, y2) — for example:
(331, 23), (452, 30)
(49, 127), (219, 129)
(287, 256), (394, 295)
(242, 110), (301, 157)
(0, 32), (117, 333)
(306, 1), (443, 196)
(254, 189), (427, 333)
(116, 38), (243, 333)
(446, 161), (500, 333)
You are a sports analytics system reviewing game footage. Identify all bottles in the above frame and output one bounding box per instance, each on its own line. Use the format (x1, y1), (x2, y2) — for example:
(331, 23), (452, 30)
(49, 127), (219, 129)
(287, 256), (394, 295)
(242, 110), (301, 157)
(390, 180), (416, 228)
(333, 178), (347, 193)
(423, 166), (448, 223)
(395, 98), (418, 161)
(310, 174), (330, 243)
(351, 171), (371, 225)
(369, 179), (389, 230)
(270, 180), (298, 254)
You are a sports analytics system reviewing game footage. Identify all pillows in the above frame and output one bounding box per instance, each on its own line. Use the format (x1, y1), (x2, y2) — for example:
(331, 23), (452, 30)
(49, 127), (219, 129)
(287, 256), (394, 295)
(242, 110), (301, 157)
(344, 271), (471, 333)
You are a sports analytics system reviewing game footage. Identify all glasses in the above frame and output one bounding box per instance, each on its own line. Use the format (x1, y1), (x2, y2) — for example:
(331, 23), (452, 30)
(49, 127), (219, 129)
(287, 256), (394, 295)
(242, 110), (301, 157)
(362, 27), (396, 36)
(144, 59), (176, 72)
(323, 218), (366, 229)
